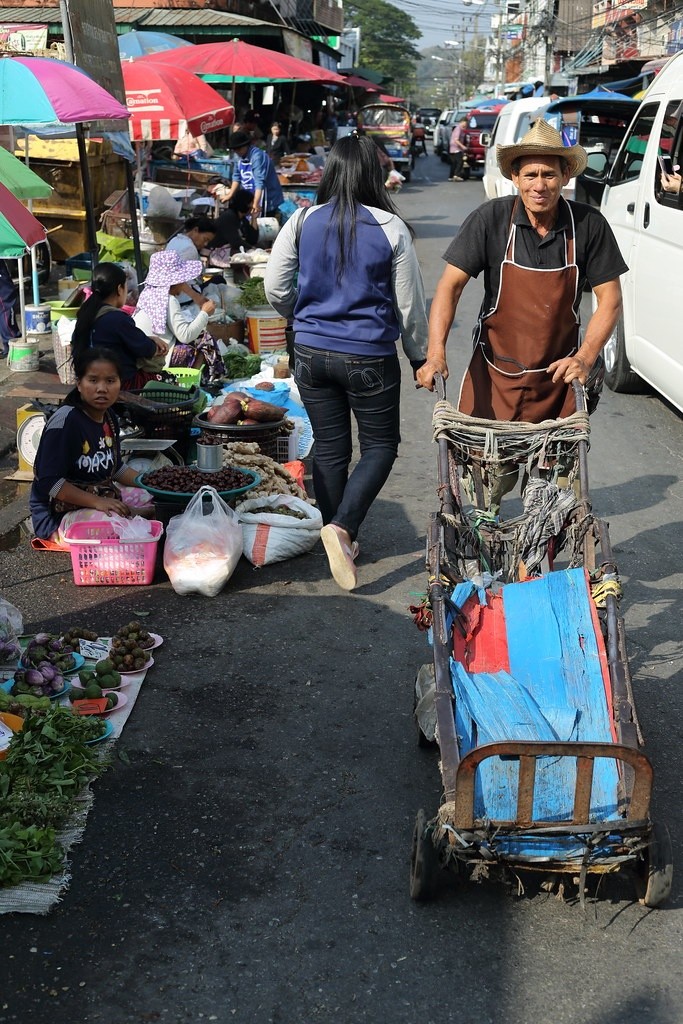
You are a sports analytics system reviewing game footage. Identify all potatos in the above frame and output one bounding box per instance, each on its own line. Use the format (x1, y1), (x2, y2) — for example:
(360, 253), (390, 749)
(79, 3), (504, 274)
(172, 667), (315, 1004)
(206, 391), (290, 426)
(255, 382), (274, 391)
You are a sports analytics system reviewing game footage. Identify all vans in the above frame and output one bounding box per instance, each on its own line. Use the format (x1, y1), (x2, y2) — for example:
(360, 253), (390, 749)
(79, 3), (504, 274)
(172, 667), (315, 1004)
(479, 96), (628, 202)
(581, 50), (683, 416)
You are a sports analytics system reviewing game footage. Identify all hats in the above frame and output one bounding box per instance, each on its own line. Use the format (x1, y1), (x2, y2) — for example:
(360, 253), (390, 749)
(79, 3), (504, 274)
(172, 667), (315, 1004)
(131, 250), (202, 335)
(228, 132), (252, 148)
(496, 117), (589, 180)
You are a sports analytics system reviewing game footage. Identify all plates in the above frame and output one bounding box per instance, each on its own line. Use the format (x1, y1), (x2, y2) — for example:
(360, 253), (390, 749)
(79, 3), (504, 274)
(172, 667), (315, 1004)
(66, 691), (128, 712)
(18, 652), (85, 673)
(96, 655), (154, 673)
(1, 678), (70, 698)
(71, 674), (130, 690)
(83, 720), (113, 743)
(109, 633), (163, 651)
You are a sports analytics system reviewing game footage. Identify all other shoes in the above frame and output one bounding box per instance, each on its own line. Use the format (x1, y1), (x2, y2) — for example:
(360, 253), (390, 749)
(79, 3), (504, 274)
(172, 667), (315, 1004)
(448, 178), (454, 181)
(454, 176), (463, 181)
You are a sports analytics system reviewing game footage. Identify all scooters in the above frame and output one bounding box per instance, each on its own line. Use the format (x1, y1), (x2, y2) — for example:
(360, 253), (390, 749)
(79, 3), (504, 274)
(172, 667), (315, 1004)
(411, 131), (425, 158)
(355, 103), (414, 183)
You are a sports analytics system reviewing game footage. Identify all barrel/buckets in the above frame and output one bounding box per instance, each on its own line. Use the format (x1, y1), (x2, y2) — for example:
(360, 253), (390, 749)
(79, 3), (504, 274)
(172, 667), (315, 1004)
(246, 305), (287, 355)
(7, 338), (40, 372)
(25, 304), (52, 334)
(249, 217), (279, 246)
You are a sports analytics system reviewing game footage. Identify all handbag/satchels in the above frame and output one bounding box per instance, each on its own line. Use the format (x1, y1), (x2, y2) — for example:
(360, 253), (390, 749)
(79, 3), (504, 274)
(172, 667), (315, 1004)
(385, 169), (406, 194)
(51, 318), (79, 384)
(162, 486), (243, 598)
(55, 482), (122, 512)
(285, 207), (309, 373)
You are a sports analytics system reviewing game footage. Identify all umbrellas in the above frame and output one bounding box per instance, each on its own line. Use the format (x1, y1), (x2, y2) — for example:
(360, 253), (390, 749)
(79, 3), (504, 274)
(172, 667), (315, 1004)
(0, 29), (232, 344)
(135, 39), (350, 126)
(338, 68), (407, 105)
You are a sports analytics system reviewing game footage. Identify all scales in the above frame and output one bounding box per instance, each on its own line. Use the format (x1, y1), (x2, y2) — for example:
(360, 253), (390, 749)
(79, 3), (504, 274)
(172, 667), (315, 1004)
(0, 371), (76, 473)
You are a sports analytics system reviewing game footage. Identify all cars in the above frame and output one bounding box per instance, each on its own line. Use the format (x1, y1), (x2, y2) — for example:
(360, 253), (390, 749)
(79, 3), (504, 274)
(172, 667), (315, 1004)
(410, 107), (442, 135)
(429, 110), (472, 162)
(452, 109), (500, 179)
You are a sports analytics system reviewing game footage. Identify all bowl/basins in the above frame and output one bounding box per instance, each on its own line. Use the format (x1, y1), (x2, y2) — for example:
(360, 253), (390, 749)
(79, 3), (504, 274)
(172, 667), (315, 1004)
(0, 712), (24, 761)
(135, 465), (262, 500)
(43, 301), (81, 320)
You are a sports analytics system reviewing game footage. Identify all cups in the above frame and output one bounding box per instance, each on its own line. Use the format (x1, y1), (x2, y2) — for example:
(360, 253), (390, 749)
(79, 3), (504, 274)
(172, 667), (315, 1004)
(196, 442), (224, 473)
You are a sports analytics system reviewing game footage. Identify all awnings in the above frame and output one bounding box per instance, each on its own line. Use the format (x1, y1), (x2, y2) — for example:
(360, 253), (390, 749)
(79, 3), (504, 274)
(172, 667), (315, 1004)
(559, 54), (668, 78)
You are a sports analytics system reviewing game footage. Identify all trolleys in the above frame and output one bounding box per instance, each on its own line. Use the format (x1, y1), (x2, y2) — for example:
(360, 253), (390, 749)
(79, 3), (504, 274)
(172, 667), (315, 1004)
(411, 373), (673, 907)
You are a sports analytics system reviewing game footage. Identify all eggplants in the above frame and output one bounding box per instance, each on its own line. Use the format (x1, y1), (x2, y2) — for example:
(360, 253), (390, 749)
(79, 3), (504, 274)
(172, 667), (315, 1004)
(0, 615), (77, 696)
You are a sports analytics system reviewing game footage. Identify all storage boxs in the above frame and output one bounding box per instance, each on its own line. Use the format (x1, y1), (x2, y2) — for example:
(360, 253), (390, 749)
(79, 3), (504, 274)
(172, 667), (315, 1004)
(150, 157), (232, 182)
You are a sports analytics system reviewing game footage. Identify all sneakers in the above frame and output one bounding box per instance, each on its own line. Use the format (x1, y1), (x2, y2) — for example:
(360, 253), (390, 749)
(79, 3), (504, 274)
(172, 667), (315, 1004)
(320, 524), (359, 591)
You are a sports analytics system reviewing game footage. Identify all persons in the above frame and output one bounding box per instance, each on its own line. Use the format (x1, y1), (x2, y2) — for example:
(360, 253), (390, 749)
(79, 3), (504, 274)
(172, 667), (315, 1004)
(264, 135), (430, 591)
(201, 189), (261, 267)
(164, 216), (229, 308)
(70, 262), (180, 398)
(29, 349), (153, 546)
(416, 116), (630, 514)
(661, 170), (682, 195)
(448, 118), (469, 182)
(220, 133), (284, 218)
(233, 101), (356, 171)
(173, 130), (215, 161)
(131, 249), (226, 395)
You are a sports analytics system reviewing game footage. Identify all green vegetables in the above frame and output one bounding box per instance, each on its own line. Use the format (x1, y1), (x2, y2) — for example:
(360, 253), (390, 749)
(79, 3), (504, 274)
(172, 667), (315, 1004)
(0, 701), (105, 887)
(222, 346), (264, 379)
(232, 276), (268, 307)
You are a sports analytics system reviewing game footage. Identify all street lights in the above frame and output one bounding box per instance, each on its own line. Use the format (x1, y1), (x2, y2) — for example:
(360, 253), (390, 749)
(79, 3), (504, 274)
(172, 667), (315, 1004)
(462, 0), (553, 97)
(432, 55), (478, 92)
(444, 40), (506, 100)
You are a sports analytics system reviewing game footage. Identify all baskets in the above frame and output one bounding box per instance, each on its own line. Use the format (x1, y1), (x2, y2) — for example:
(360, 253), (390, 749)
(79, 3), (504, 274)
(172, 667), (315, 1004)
(113, 384), (199, 464)
(63, 520), (163, 584)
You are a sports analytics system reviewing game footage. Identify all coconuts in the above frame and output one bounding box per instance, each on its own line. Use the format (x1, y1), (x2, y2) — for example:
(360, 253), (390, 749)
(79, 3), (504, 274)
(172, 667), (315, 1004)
(273, 356), (291, 379)
